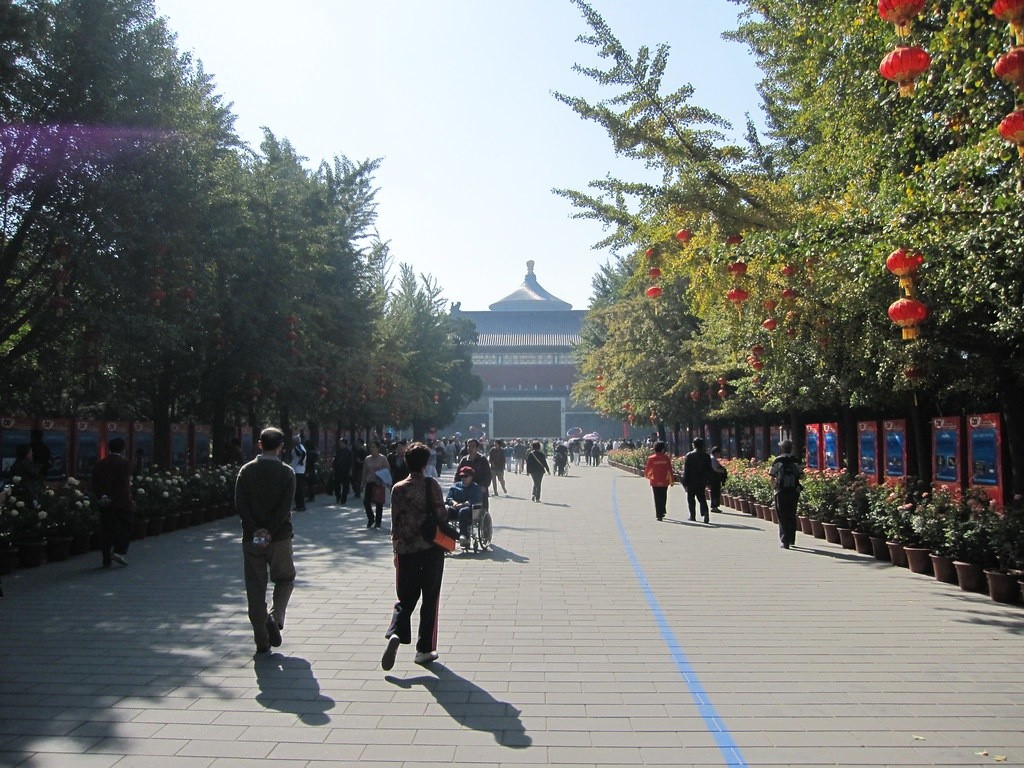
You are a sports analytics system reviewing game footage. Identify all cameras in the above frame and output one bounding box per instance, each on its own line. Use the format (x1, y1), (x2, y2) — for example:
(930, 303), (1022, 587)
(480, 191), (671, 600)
(252, 536), (266, 544)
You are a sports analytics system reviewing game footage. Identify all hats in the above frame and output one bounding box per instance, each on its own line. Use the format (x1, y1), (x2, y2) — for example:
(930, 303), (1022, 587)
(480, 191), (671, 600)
(459, 466), (474, 476)
(778, 440), (793, 451)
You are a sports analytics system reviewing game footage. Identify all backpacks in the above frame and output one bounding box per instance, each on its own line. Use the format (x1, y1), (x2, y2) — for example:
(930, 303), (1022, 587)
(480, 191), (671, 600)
(777, 457), (799, 492)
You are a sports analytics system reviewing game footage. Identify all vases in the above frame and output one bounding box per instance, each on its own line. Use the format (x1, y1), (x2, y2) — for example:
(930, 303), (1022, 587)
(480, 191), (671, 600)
(166, 514), (179, 532)
(798, 516), (812, 534)
(732, 496), (742, 510)
(16, 542), (48, 566)
(809, 519), (824, 538)
(726, 494), (733, 507)
(149, 517), (166, 535)
(760, 505), (771, 522)
(0, 548), (18, 575)
(754, 503), (762, 517)
(178, 511), (192, 528)
(837, 527), (854, 549)
(769, 507), (779, 524)
(137, 519), (149, 538)
(852, 531), (1024, 604)
(46, 537), (75, 561)
(739, 498), (748, 512)
(74, 531), (93, 553)
(821, 522), (837, 542)
(747, 501), (755, 514)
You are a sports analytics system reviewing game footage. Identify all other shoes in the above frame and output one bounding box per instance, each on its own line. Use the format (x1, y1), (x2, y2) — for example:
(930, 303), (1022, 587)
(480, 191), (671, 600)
(459, 535), (467, 547)
(537, 500), (541, 503)
(532, 495), (535, 501)
(711, 508), (721, 512)
(415, 652), (437, 664)
(375, 519), (381, 528)
(367, 518), (374, 527)
(293, 507), (306, 511)
(257, 643), (270, 656)
(104, 562), (112, 568)
(504, 489), (507, 493)
(492, 493), (498, 496)
(781, 544), (790, 548)
(382, 634), (399, 671)
(268, 615), (282, 647)
(704, 514), (708, 523)
(110, 553), (128, 566)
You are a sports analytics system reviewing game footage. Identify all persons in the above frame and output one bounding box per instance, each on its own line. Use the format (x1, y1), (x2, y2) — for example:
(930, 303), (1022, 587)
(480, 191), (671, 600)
(768, 440), (804, 549)
(360, 440), (393, 529)
(381, 442), (450, 671)
(644, 440), (674, 521)
(290, 434), (307, 512)
(444, 466), (483, 547)
(708, 445), (728, 514)
(225, 437), (243, 464)
(682, 436), (712, 524)
(254, 431), (667, 506)
(453, 437), (492, 526)
(90, 437), (133, 570)
(234, 426), (297, 658)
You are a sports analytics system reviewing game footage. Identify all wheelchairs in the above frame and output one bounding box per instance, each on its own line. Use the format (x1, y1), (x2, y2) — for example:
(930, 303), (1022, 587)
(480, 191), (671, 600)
(447, 489), (494, 553)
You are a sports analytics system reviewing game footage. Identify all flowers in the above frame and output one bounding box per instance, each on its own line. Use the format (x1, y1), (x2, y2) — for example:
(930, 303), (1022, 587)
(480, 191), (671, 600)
(0, 456), (239, 550)
(725, 449), (1024, 570)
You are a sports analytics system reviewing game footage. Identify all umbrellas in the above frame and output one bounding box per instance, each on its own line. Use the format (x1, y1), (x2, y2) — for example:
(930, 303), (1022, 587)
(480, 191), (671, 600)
(583, 434), (599, 440)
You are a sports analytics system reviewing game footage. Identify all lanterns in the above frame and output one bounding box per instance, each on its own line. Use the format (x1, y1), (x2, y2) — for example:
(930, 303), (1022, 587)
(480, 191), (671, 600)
(879, 41), (931, 101)
(878, 0), (926, 37)
(594, 228), (924, 430)
(991, 0), (1024, 159)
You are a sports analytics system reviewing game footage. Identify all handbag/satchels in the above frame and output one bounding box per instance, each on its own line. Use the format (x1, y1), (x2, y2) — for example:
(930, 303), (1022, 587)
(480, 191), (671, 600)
(371, 478), (385, 504)
(423, 476), (456, 551)
